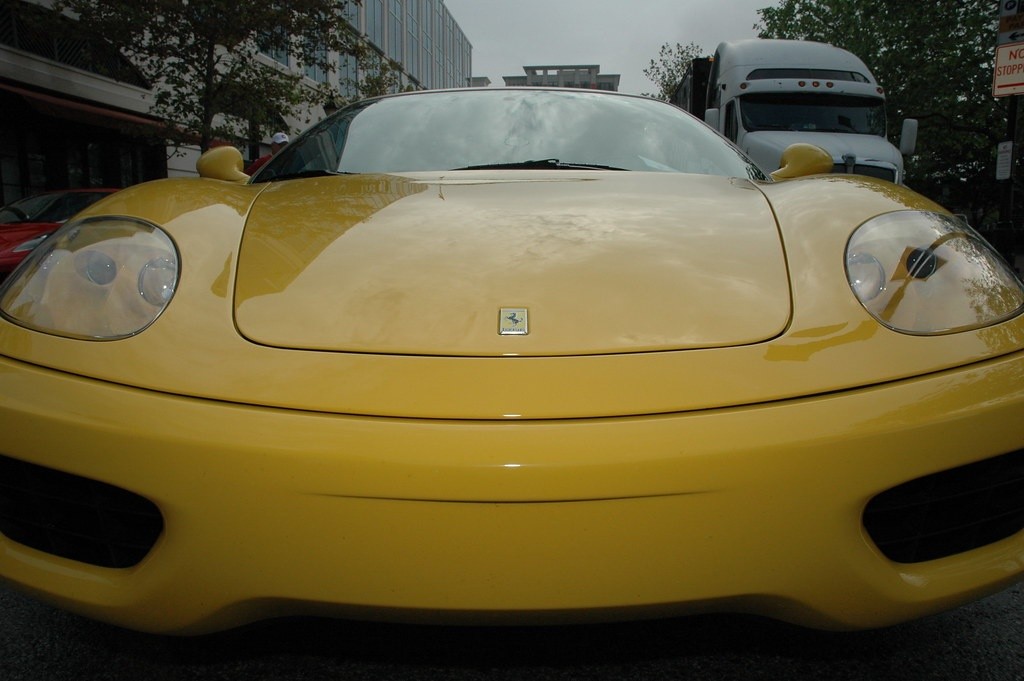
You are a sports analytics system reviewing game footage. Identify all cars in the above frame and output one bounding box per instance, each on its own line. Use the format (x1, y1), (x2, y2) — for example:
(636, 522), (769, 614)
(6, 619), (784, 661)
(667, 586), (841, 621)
(0, 188), (121, 274)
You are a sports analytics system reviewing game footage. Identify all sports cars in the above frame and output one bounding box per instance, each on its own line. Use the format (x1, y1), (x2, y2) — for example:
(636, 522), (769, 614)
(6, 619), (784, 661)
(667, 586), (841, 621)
(0, 86), (1023, 681)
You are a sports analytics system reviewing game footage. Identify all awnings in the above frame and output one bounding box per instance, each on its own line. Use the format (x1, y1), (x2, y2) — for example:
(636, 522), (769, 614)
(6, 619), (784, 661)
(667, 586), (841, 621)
(0, 84), (235, 151)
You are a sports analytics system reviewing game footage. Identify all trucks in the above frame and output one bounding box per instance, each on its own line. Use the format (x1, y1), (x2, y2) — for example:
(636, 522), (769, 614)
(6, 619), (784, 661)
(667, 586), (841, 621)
(672, 40), (905, 184)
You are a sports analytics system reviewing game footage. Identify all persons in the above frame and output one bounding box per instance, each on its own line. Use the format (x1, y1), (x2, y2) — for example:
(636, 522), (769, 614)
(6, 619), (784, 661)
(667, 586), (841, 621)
(243, 132), (290, 176)
